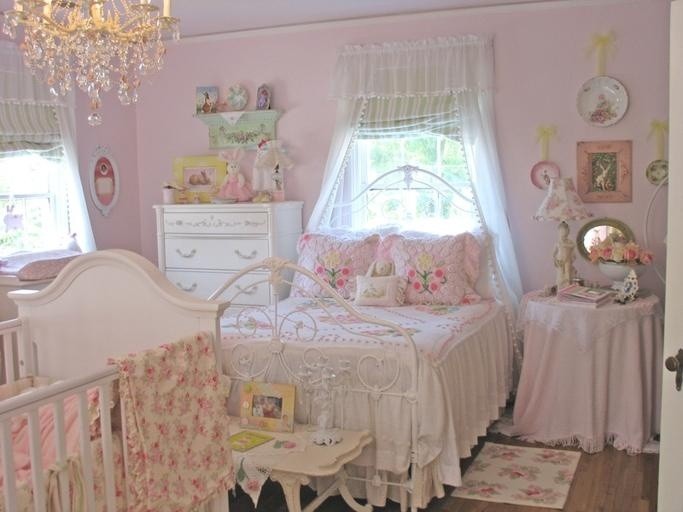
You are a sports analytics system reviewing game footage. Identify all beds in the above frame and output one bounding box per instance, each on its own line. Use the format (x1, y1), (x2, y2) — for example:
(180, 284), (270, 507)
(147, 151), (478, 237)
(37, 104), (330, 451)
(0, 251), (232, 510)
(204, 258), (508, 510)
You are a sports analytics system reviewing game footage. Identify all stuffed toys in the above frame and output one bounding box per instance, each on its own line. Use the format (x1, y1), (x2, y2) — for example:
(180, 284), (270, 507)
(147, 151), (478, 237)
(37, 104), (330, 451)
(366, 258), (395, 277)
(215, 147), (257, 203)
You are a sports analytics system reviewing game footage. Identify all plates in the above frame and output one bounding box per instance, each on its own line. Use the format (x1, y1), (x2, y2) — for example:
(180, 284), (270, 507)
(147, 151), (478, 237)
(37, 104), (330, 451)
(528, 160), (562, 191)
(644, 158), (669, 186)
(225, 83), (249, 111)
(255, 84), (271, 110)
(575, 74), (631, 129)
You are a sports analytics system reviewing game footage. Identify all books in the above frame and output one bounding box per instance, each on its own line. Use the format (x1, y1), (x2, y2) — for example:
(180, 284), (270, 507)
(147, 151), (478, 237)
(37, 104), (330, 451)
(558, 283), (613, 303)
(558, 295), (613, 308)
(228, 429), (275, 452)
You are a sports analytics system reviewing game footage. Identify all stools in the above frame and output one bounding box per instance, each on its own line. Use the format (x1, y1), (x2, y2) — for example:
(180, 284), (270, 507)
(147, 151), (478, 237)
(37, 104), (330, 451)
(228, 412), (372, 511)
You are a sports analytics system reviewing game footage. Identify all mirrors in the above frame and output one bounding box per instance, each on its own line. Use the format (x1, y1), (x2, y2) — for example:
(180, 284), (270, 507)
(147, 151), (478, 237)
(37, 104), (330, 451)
(579, 218), (636, 266)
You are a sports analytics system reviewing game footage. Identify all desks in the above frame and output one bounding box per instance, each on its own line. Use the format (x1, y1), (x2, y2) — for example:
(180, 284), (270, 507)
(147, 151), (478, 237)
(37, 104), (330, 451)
(525, 289), (659, 443)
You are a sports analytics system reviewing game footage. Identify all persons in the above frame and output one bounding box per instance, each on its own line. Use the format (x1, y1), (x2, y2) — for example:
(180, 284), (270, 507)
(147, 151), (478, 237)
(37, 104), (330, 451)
(262, 396), (280, 419)
(252, 395), (265, 417)
(553, 222), (577, 284)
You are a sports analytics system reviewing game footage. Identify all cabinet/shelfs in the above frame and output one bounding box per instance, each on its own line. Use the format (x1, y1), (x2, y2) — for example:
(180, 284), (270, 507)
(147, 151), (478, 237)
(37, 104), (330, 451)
(151, 201), (305, 319)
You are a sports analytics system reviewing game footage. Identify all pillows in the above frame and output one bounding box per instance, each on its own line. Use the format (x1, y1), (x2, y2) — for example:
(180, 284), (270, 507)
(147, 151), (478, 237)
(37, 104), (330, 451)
(295, 227), (486, 307)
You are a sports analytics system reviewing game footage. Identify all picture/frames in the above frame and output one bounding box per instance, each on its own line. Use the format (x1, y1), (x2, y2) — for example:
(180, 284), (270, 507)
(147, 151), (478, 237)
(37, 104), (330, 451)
(88, 144), (119, 216)
(171, 154), (226, 205)
(238, 380), (295, 432)
(575, 139), (632, 203)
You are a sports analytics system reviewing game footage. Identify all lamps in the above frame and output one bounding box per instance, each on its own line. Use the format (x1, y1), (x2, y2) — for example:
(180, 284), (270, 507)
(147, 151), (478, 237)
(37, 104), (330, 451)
(0, 1), (182, 123)
(531, 177), (595, 290)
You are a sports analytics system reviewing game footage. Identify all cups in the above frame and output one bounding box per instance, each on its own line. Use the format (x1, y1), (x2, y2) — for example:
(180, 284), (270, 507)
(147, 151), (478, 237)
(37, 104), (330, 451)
(162, 188), (178, 205)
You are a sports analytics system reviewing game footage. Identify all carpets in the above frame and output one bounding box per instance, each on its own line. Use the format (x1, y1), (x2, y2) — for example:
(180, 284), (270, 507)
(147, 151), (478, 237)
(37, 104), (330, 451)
(451, 441), (581, 508)
